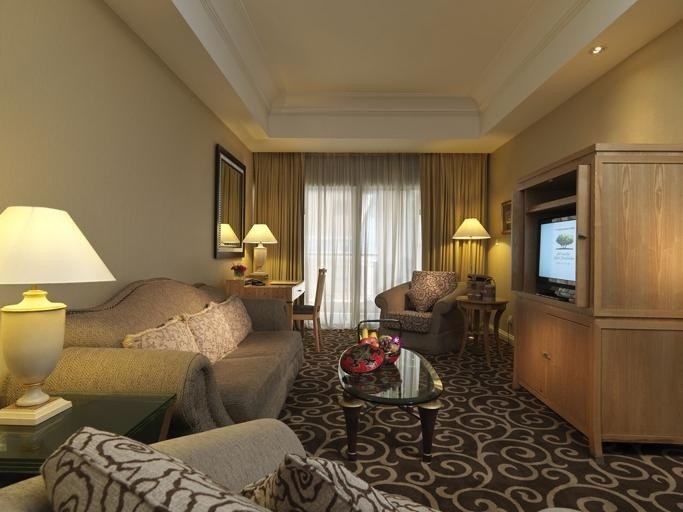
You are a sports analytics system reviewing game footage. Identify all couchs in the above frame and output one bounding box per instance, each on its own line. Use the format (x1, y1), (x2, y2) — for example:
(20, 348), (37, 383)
(1, 275), (306, 434)
(0, 418), (445, 512)
(375, 281), (467, 356)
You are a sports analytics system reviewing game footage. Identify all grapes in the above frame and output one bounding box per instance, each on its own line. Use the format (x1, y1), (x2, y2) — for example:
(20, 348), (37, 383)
(379, 339), (392, 353)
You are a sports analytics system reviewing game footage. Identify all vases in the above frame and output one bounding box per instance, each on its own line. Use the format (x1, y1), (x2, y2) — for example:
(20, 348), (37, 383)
(234, 271), (244, 277)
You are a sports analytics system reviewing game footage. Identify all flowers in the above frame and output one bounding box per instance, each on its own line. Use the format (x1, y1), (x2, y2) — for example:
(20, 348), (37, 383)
(231, 264), (247, 272)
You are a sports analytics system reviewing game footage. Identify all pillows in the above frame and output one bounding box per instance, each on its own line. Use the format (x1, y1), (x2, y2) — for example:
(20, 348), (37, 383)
(124, 317), (201, 355)
(213, 293), (253, 346)
(405, 270), (456, 313)
(240, 450), (443, 512)
(185, 302), (238, 362)
(39, 427), (267, 511)
(404, 277), (446, 312)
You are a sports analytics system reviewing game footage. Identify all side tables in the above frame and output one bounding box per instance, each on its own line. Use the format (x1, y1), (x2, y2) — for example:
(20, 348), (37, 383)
(455, 295), (509, 369)
(0, 392), (177, 484)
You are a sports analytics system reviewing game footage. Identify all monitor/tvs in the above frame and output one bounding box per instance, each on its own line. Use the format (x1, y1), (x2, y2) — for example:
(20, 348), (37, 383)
(537, 216), (576, 289)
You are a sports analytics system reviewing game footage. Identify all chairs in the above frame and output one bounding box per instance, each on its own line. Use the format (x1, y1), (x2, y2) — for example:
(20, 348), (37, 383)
(293, 268), (327, 352)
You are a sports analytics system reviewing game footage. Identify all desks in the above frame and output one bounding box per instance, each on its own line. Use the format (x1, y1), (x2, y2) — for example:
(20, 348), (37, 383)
(225, 276), (306, 339)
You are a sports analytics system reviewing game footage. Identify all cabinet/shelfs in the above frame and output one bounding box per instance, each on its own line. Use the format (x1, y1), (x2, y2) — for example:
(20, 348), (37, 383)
(510, 140), (682, 462)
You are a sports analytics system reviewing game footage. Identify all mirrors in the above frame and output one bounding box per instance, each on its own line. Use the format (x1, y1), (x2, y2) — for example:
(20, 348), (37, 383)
(214, 143), (247, 259)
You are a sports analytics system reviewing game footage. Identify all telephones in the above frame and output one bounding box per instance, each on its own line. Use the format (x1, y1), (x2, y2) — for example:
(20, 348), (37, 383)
(250, 278), (265, 286)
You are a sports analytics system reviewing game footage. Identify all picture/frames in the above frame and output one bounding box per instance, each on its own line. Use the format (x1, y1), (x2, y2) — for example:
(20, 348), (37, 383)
(501, 200), (514, 235)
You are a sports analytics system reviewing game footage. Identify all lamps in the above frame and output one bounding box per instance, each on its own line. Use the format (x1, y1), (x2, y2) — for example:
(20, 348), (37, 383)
(243, 224), (278, 281)
(0, 206), (117, 426)
(451, 219), (492, 275)
(220, 224), (241, 246)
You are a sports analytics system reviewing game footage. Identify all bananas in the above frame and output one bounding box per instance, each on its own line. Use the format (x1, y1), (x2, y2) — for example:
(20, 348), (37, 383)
(362, 326), (368, 339)
